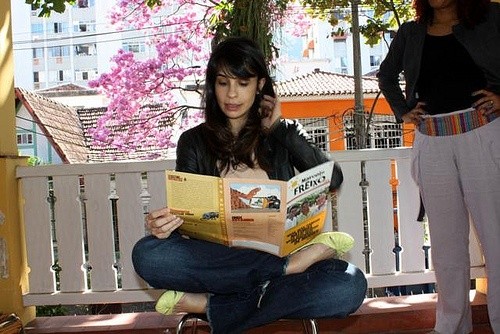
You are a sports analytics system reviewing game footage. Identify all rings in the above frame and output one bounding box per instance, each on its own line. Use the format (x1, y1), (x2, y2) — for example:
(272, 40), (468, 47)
(489, 100), (495, 106)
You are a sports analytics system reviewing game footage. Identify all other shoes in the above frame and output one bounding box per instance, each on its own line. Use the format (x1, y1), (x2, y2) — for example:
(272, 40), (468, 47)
(153, 289), (184, 316)
(288, 231), (355, 261)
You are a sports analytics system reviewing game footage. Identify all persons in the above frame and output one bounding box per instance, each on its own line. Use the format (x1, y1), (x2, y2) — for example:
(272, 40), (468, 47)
(374, 0), (500, 334)
(131, 35), (368, 334)
